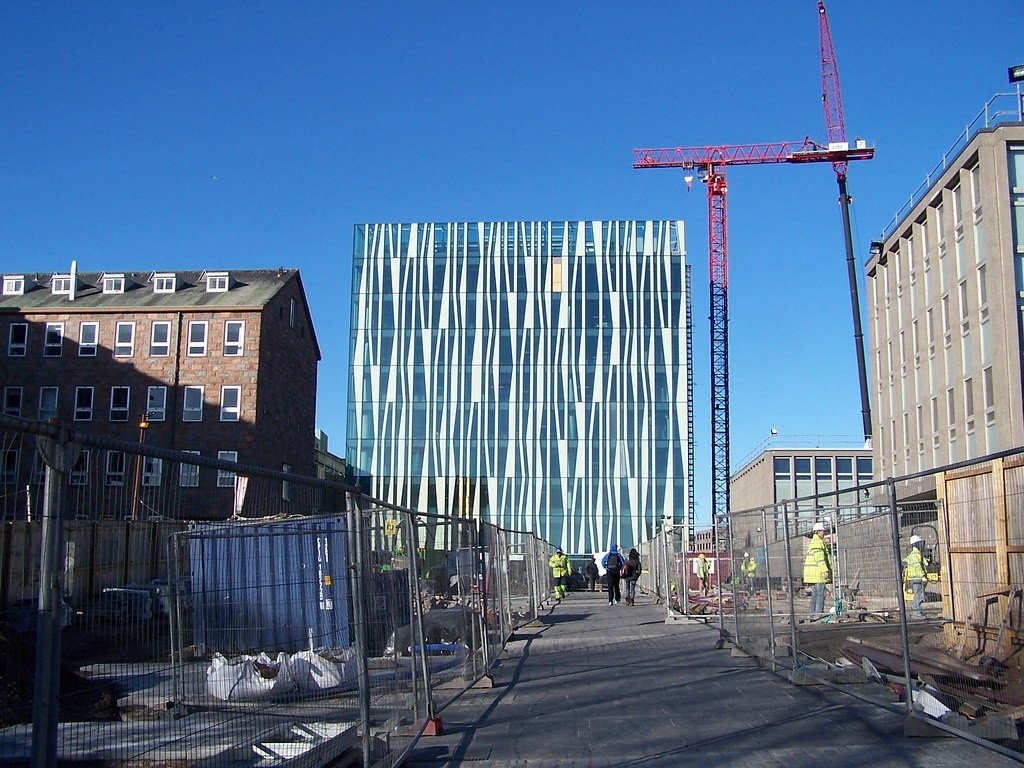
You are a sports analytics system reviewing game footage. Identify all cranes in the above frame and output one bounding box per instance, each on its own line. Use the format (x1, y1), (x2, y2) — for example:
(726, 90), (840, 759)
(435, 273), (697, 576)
(632, 132), (875, 536)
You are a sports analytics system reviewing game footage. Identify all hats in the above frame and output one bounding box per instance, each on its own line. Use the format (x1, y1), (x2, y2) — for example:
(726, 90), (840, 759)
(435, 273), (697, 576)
(591, 558), (596, 561)
(556, 548), (562, 552)
(744, 552), (750, 558)
(910, 535), (922, 544)
(813, 523), (827, 530)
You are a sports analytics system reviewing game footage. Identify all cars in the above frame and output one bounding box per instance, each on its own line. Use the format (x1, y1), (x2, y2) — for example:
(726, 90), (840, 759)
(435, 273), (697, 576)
(8, 592), (78, 631)
(83, 575), (194, 625)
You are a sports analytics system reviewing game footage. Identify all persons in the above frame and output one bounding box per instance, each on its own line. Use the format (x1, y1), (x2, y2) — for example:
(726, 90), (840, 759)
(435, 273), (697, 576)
(804, 522), (832, 622)
(907, 535), (929, 620)
(623, 548), (641, 606)
(804, 136), (810, 153)
(602, 544), (624, 605)
(741, 552), (757, 594)
(586, 558), (599, 592)
(549, 548), (572, 605)
(697, 554), (710, 595)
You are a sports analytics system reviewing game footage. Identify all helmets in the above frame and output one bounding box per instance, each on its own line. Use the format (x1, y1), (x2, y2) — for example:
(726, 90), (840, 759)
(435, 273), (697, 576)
(698, 553), (705, 559)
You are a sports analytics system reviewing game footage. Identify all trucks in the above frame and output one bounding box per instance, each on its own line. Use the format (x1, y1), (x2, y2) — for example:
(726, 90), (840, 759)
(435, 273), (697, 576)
(506, 554), (596, 593)
(198, 512), (415, 657)
(419, 546), (526, 595)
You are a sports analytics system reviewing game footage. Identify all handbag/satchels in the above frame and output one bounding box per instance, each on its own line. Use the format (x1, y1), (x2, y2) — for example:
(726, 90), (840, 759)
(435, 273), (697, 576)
(619, 564), (628, 579)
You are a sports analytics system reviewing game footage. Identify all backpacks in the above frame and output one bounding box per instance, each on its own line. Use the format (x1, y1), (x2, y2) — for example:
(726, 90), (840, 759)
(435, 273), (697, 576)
(588, 569), (594, 574)
(607, 552), (620, 570)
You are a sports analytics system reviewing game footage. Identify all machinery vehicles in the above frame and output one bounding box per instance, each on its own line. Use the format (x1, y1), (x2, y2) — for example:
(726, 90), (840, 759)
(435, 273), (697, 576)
(815, 2), (877, 449)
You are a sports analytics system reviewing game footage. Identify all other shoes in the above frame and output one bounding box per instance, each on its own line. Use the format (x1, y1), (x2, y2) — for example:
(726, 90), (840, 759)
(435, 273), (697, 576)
(608, 601), (613, 606)
(562, 594), (565, 598)
(591, 590), (595, 592)
(630, 599), (635, 606)
(615, 600), (617, 605)
(556, 598), (560, 601)
(626, 598), (630, 605)
